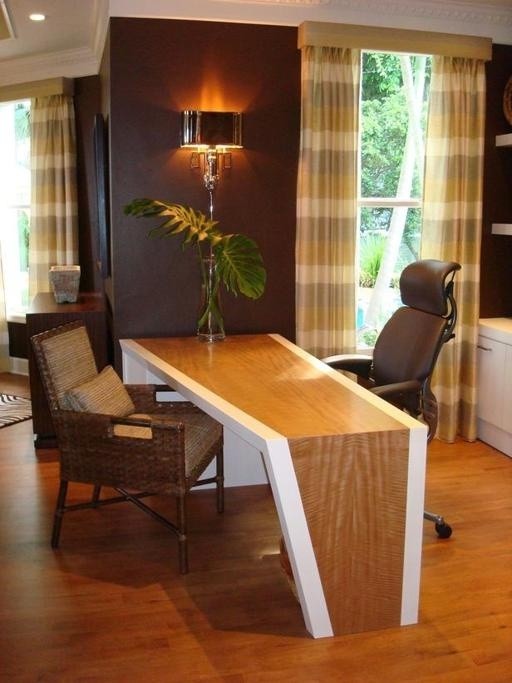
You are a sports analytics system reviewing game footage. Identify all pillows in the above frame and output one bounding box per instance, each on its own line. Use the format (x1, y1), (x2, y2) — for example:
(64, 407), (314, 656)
(65, 363), (138, 418)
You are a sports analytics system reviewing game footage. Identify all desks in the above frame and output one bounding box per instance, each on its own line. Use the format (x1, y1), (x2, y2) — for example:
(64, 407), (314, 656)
(117, 332), (429, 642)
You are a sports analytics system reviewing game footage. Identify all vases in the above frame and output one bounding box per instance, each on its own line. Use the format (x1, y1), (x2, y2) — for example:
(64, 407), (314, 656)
(47, 264), (83, 304)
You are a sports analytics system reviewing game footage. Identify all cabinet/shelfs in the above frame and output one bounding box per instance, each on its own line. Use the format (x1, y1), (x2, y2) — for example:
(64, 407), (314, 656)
(474, 131), (512, 459)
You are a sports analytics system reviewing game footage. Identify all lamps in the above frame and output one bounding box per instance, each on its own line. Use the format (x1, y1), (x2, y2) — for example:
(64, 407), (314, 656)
(179, 108), (244, 333)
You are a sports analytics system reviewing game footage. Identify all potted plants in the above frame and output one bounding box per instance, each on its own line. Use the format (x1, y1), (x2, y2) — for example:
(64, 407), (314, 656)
(121, 196), (267, 343)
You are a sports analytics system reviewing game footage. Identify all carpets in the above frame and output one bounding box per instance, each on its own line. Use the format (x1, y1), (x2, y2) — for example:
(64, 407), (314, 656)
(0, 391), (34, 431)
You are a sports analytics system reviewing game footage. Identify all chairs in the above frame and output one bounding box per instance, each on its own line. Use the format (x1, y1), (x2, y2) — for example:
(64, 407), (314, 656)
(317, 257), (463, 446)
(30, 319), (224, 576)
